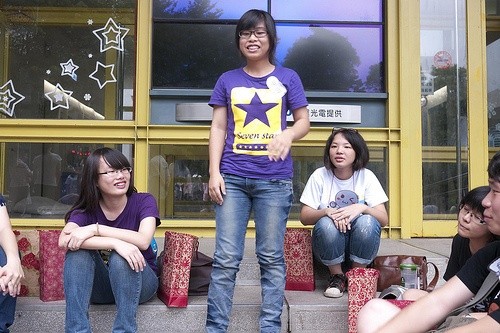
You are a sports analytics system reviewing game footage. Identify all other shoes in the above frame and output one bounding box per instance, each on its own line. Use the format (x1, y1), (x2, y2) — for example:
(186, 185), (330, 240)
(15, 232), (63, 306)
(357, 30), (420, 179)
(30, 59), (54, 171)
(323, 273), (348, 298)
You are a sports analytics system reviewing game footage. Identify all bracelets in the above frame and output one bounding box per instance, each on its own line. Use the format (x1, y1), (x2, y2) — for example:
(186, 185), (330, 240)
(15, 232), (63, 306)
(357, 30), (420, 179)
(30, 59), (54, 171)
(96, 221), (102, 236)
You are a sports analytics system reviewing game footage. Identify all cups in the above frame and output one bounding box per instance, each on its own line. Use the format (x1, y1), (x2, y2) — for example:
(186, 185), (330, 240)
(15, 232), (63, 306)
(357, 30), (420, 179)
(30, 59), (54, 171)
(399, 263), (421, 291)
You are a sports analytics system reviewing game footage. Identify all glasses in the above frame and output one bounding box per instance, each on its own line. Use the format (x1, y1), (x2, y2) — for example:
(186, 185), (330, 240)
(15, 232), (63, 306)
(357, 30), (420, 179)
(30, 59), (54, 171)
(238, 28), (269, 38)
(332, 126), (359, 134)
(459, 203), (487, 225)
(97, 166), (134, 176)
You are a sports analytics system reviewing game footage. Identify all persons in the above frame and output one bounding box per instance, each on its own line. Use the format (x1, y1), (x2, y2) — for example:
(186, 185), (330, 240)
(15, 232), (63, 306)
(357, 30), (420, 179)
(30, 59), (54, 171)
(353, 149), (499, 333)
(0, 190), (26, 333)
(299, 126), (390, 299)
(401, 185), (500, 314)
(59, 146), (162, 333)
(5, 141), (211, 217)
(203, 10), (312, 333)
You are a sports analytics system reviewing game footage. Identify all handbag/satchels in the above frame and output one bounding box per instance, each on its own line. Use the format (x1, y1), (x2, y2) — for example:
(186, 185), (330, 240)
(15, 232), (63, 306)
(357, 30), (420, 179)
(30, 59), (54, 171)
(157, 250), (215, 296)
(38, 228), (66, 302)
(13, 230), (40, 298)
(157, 231), (199, 308)
(370, 254), (439, 292)
